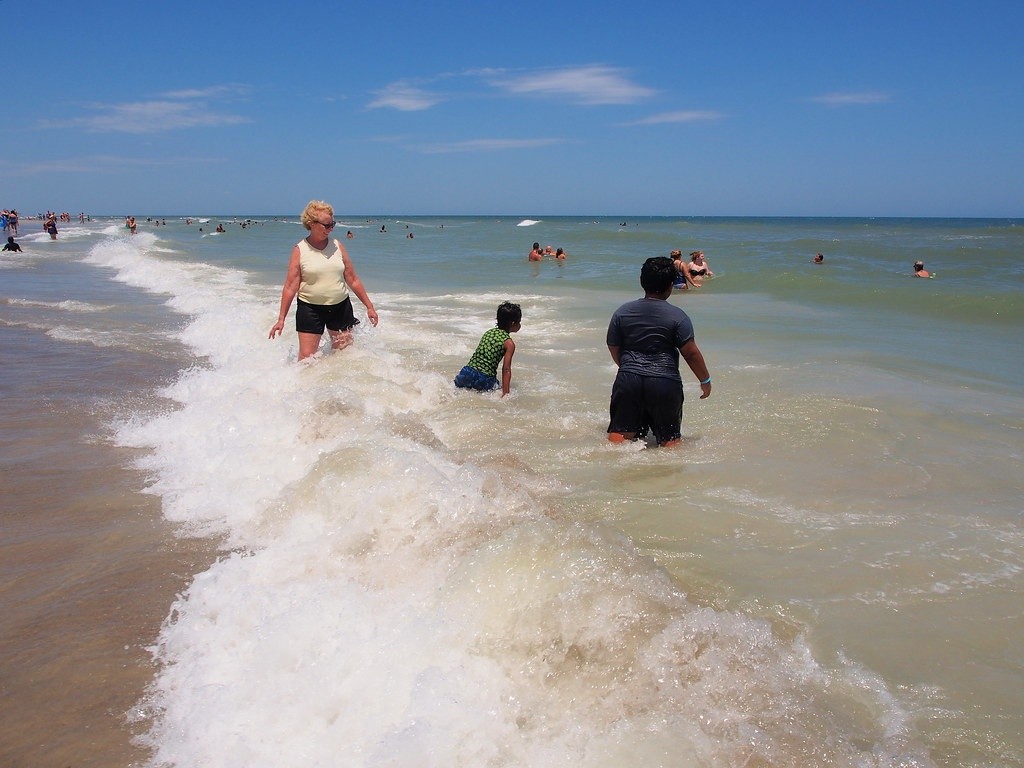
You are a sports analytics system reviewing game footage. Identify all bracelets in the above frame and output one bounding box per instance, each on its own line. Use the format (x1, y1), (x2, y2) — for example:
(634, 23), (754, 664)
(700, 377), (711, 385)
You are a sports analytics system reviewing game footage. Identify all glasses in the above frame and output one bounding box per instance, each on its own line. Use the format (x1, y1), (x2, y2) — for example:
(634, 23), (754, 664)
(316, 221), (336, 229)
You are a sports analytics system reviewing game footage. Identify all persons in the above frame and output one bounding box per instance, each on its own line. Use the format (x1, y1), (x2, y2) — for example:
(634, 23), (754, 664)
(455, 300), (522, 400)
(542, 246), (556, 255)
(1, 236), (23, 252)
(670, 249), (702, 289)
(607, 257), (712, 447)
(529, 243), (541, 262)
(1, 209), (447, 242)
(814, 253), (824, 263)
(687, 251), (713, 280)
(556, 247), (565, 259)
(537, 248), (543, 255)
(268, 199), (379, 362)
(913, 260), (929, 278)
